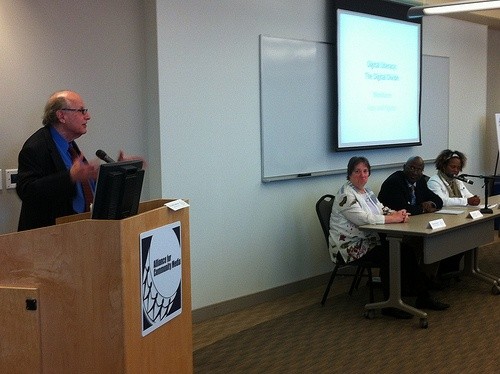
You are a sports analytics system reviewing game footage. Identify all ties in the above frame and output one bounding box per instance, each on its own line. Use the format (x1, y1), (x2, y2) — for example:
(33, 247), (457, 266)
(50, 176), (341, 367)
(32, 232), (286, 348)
(68, 146), (93, 204)
(410, 185), (417, 205)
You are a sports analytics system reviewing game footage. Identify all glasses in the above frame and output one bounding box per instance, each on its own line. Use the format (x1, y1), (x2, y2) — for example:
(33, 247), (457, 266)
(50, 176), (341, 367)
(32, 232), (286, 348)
(56, 108), (89, 115)
(405, 165), (424, 173)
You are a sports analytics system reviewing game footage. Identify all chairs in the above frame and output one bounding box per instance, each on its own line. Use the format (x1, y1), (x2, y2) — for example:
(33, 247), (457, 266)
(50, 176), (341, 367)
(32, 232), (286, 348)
(316, 194), (383, 308)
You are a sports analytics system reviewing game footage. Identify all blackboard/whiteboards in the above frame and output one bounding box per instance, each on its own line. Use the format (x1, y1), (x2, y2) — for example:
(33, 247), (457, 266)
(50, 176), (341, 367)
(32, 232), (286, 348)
(260, 34), (450, 182)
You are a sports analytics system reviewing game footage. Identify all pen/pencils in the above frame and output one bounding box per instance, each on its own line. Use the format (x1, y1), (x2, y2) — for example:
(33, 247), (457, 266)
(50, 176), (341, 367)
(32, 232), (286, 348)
(388, 212), (411, 215)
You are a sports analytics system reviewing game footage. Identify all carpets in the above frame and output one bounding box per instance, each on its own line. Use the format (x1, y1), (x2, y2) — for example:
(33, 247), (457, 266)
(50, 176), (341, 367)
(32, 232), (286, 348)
(193, 241), (500, 374)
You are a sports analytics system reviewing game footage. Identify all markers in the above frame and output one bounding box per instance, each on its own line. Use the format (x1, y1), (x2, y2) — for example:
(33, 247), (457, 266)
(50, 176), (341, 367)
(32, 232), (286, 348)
(296, 173), (311, 177)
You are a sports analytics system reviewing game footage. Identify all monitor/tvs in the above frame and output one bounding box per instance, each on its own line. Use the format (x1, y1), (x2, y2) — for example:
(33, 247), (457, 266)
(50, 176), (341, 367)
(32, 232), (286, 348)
(90, 159), (146, 220)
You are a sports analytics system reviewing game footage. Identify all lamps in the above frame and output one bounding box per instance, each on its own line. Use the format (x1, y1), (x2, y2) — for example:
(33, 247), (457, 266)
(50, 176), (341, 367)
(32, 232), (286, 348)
(407, 0), (500, 18)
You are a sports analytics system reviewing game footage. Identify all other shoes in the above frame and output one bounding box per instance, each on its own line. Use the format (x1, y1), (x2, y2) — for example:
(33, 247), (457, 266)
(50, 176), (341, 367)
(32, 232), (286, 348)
(382, 307), (414, 319)
(415, 295), (450, 311)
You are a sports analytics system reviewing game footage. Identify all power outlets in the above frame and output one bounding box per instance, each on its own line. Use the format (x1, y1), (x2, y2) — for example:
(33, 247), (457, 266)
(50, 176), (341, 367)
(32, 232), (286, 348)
(5, 169), (18, 189)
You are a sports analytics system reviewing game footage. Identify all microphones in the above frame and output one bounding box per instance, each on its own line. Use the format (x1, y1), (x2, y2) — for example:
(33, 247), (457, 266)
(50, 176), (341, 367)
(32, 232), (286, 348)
(448, 173), (473, 185)
(95, 149), (114, 163)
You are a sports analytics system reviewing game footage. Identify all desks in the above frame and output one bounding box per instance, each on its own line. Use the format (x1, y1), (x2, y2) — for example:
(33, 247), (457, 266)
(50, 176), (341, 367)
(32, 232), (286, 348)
(358, 194), (500, 328)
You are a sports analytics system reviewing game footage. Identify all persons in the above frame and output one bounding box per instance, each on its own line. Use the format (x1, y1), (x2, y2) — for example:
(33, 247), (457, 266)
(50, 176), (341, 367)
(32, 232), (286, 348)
(328, 156), (409, 318)
(378, 158), (450, 310)
(17, 90), (145, 232)
(427, 149), (472, 200)
